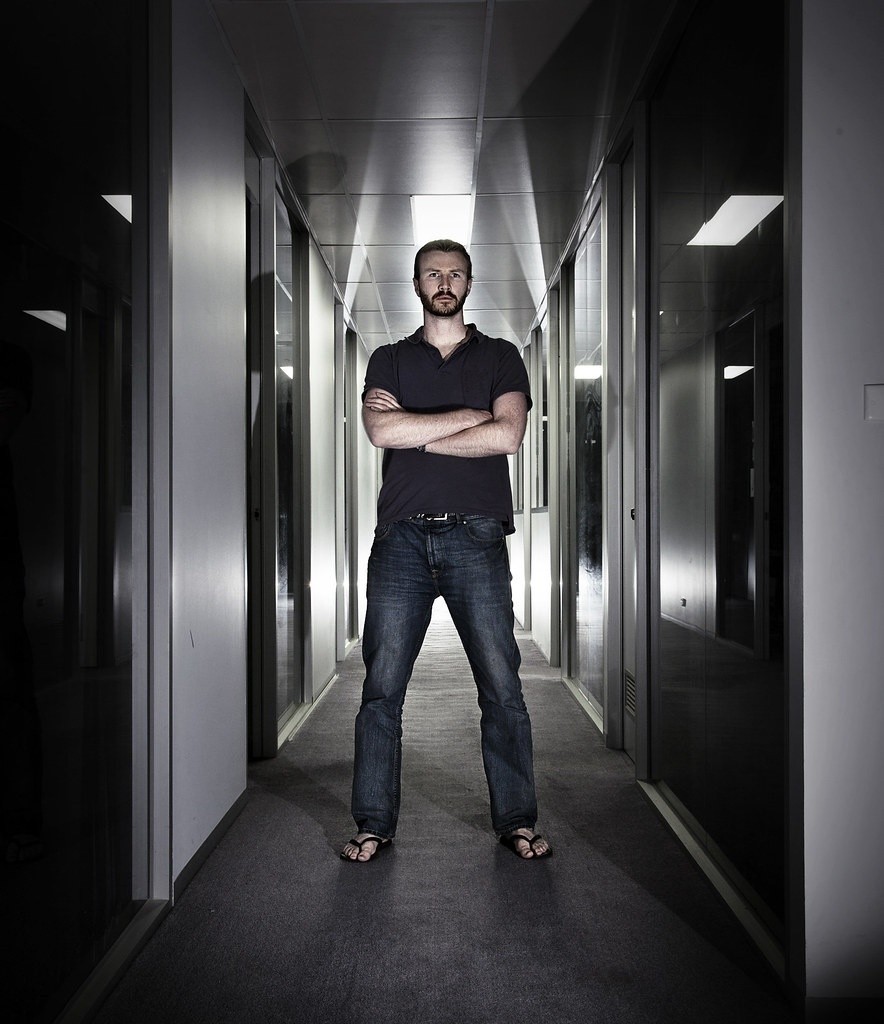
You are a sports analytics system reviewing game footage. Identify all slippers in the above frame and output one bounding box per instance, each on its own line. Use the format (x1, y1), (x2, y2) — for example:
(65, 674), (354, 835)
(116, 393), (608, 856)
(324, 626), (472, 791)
(499, 831), (553, 859)
(339, 839), (392, 863)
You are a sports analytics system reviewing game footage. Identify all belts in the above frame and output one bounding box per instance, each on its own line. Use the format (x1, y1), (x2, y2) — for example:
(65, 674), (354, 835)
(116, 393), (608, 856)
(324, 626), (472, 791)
(402, 512), (474, 521)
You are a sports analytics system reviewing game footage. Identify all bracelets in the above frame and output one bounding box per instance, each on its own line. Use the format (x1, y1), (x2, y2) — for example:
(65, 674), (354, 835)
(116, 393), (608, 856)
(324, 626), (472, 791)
(417, 445), (426, 453)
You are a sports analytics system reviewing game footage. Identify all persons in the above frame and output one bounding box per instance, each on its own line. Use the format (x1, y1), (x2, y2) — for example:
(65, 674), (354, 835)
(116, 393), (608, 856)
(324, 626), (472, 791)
(340, 240), (551, 862)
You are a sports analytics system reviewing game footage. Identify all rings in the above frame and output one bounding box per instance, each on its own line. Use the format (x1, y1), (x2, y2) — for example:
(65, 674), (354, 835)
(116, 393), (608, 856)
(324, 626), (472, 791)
(388, 409), (392, 412)
